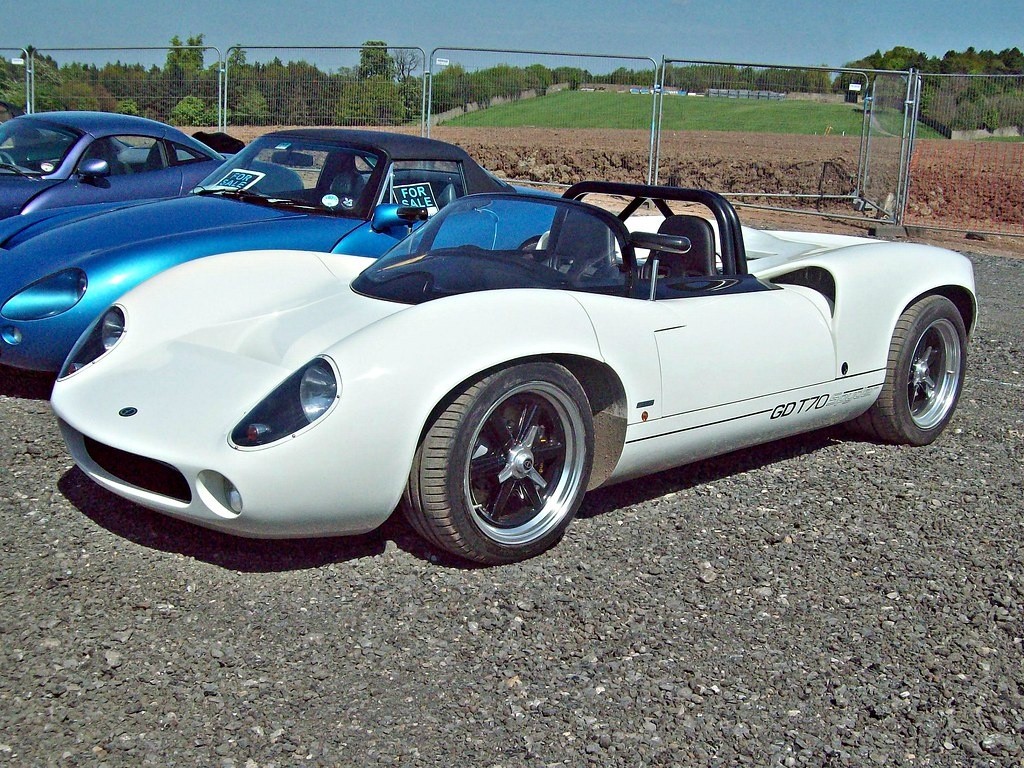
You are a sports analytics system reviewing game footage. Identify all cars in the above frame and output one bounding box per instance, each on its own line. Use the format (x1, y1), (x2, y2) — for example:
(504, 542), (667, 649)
(630, 89), (640, 95)
(581, 87), (594, 91)
(640, 89), (649, 94)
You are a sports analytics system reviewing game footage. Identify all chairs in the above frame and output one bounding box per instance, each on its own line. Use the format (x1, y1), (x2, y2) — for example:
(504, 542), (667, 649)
(425, 177), (458, 210)
(556, 209), (617, 277)
(10, 128), (57, 171)
(642, 215), (717, 280)
(79, 138), (133, 177)
(320, 170), (367, 211)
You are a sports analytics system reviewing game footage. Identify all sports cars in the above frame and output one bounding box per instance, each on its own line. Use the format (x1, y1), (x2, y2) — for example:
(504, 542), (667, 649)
(49, 179), (980, 566)
(0, 111), (305, 222)
(0, 130), (564, 373)
